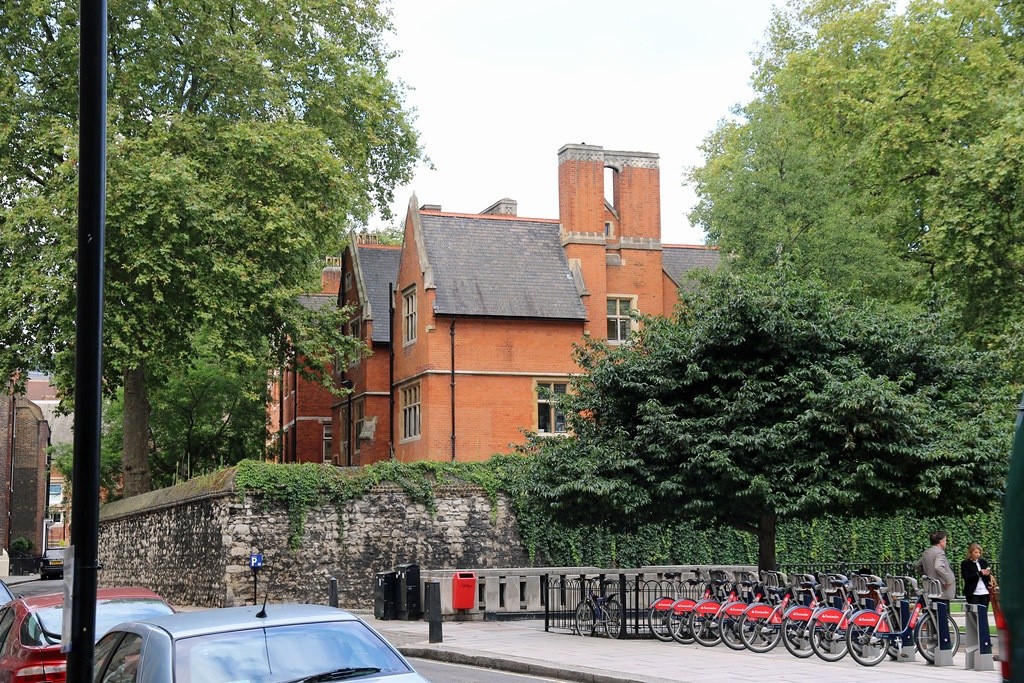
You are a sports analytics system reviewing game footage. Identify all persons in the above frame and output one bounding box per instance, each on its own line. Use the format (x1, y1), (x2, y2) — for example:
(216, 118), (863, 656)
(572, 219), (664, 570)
(961, 545), (994, 654)
(914, 531), (955, 650)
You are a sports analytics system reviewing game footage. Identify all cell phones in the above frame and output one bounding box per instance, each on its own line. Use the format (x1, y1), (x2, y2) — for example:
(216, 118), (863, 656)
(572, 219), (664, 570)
(987, 568), (991, 572)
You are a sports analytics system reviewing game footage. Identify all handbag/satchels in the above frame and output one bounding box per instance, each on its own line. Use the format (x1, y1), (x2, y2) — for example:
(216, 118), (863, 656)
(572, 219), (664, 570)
(987, 570), (998, 587)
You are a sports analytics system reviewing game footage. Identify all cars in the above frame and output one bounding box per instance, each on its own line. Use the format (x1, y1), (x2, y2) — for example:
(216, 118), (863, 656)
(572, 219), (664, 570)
(93, 601), (431, 682)
(0, 584), (178, 683)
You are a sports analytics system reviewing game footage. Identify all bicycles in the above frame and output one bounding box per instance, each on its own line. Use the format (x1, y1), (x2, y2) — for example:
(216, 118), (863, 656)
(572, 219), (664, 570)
(573, 577), (624, 639)
(645, 569), (960, 667)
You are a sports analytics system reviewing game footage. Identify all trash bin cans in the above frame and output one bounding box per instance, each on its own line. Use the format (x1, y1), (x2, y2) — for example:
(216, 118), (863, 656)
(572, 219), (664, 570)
(452, 571), (476, 609)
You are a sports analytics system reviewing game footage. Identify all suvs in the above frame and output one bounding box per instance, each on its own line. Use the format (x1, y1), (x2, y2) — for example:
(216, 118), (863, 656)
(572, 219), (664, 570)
(40, 547), (65, 580)
